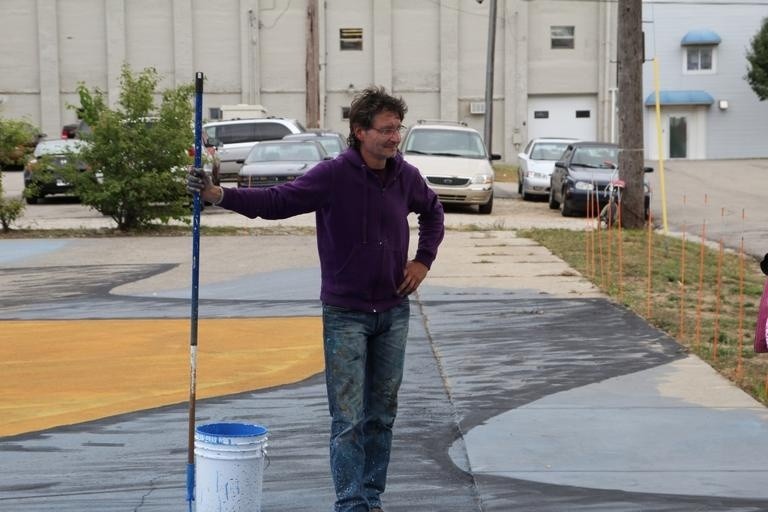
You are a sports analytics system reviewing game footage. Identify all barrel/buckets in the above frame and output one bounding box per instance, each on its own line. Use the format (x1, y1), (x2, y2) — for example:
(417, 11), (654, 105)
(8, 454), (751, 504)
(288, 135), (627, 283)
(191, 421), (271, 512)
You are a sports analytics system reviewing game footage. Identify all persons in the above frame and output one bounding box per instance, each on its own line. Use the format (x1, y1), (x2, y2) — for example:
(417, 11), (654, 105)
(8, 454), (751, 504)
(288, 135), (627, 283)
(186, 84), (449, 511)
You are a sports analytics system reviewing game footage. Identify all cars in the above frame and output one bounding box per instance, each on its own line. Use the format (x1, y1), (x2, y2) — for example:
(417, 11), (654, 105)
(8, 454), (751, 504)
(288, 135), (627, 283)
(59, 121), (88, 141)
(0, 123), (48, 169)
(113, 116), (196, 128)
(237, 139), (334, 189)
(548, 142), (654, 220)
(516, 135), (585, 201)
(23, 137), (101, 205)
(282, 131), (348, 161)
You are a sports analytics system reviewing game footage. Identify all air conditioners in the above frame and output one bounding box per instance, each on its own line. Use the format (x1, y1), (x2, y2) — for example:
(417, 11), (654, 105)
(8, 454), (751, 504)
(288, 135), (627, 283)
(469, 100), (486, 115)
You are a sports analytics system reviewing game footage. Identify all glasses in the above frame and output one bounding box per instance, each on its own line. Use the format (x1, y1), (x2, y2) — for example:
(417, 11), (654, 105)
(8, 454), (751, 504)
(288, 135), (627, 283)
(373, 123), (407, 136)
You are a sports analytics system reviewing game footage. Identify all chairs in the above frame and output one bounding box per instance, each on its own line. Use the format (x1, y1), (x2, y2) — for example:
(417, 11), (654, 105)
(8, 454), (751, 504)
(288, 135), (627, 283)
(576, 149), (590, 165)
(532, 148), (549, 159)
(322, 142), (340, 154)
(260, 151), (280, 161)
(293, 148), (312, 160)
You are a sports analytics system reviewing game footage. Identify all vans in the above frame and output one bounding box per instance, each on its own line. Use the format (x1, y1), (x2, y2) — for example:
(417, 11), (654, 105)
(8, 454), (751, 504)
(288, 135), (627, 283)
(399, 120), (502, 214)
(95, 117), (220, 216)
(191, 117), (307, 181)
